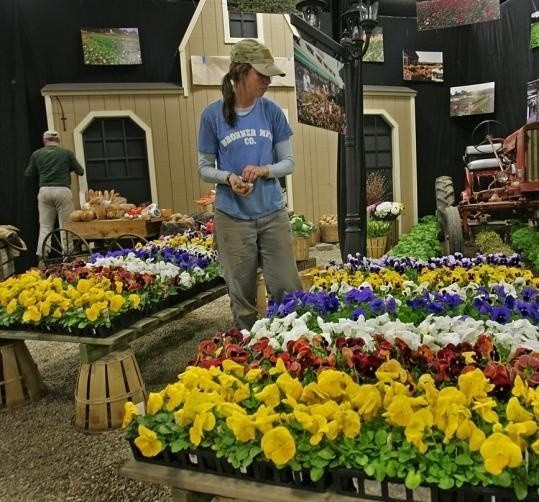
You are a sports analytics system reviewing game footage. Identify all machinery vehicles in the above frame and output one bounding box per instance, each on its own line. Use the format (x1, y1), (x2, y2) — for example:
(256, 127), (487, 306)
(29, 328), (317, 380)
(437, 120), (539, 256)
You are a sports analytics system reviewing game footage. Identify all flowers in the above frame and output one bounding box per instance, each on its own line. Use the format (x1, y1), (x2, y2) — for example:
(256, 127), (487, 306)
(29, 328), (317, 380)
(368, 202), (407, 217)
(0, 227), (214, 331)
(120, 247), (539, 502)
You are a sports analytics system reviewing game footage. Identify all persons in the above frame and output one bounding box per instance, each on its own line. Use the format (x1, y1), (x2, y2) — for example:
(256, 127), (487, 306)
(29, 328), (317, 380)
(23, 130), (84, 259)
(196, 38), (305, 334)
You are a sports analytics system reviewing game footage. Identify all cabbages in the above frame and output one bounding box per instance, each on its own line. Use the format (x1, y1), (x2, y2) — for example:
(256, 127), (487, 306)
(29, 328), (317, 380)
(289, 214), (312, 239)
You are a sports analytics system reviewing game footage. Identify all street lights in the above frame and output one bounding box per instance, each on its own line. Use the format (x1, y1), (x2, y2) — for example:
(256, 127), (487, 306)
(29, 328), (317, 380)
(338, 0), (380, 258)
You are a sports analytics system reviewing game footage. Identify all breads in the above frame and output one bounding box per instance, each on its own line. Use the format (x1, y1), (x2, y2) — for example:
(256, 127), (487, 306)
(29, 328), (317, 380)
(70, 188), (127, 221)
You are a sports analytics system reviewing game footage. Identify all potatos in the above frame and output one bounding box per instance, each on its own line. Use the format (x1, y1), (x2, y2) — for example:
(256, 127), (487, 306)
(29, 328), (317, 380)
(169, 213), (193, 222)
(318, 214), (337, 225)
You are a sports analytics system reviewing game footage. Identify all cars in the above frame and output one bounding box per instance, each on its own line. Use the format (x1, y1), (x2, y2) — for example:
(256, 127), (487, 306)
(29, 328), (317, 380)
(432, 66), (443, 79)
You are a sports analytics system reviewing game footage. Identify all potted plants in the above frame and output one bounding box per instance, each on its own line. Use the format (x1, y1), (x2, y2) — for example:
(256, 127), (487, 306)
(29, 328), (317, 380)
(365, 220), (390, 262)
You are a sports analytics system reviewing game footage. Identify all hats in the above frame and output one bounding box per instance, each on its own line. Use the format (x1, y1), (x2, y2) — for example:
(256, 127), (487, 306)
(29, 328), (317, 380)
(230, 38), (286, 77)
(41, 131), (60, 140)
